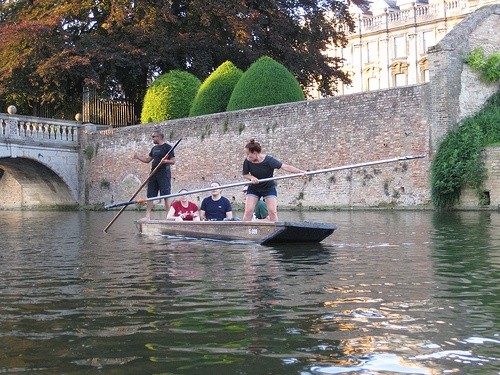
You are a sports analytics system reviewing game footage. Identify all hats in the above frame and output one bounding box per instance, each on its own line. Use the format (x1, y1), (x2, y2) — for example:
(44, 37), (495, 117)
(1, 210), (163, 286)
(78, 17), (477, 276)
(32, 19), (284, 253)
(242, 186), (248, 190)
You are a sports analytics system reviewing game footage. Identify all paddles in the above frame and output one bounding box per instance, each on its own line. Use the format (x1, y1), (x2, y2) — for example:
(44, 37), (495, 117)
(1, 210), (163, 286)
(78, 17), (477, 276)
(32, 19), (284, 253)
(103, 139), (426, 232)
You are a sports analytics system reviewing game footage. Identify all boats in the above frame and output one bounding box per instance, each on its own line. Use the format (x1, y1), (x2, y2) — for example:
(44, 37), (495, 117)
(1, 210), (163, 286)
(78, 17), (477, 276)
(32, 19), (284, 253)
(133, 218), (338, 244)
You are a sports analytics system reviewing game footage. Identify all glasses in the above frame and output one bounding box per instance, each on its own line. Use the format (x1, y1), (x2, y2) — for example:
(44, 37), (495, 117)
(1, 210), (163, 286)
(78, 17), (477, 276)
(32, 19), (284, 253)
(152, 136), (159, 138)
(242, 190), (246, 193)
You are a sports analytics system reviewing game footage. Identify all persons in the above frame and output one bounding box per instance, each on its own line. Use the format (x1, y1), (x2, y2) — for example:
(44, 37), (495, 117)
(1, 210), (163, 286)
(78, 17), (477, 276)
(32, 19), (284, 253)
(241, 138), (307, 222)
(199, 181), (233, 221)
(241, 184), (270, 220)
(131, 128), (175, 220)
(166, 188), (200, 221)
